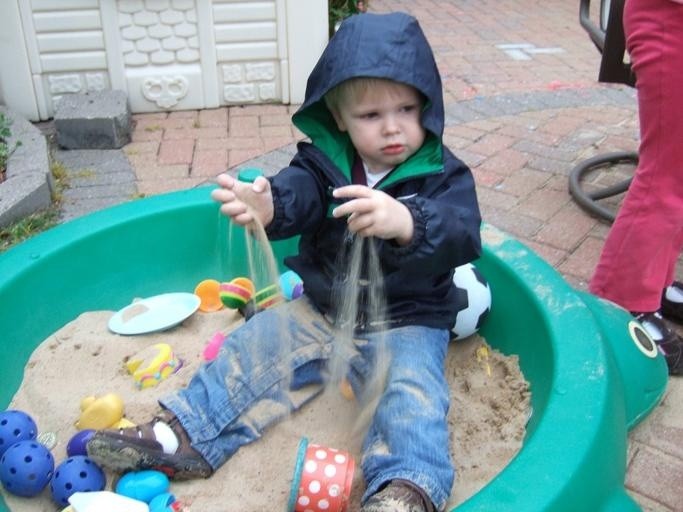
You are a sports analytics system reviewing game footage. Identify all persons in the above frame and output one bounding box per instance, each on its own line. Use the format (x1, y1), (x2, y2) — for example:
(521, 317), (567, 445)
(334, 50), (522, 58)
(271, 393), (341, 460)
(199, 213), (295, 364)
(583, 0), (681, 379)
(84, 11), (483, 512)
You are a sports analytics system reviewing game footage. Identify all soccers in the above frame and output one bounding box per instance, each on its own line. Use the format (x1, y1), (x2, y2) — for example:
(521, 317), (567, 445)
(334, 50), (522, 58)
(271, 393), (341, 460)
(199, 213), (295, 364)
(449, 263), (492, 342)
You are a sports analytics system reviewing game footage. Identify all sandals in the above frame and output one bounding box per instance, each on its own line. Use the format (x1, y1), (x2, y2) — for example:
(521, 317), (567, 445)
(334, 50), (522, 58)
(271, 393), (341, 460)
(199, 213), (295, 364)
(85, 408), (214, 481)
(660, 279), (683, 317)
(361, 479), (434, 512)
(626, 310), (682, 374)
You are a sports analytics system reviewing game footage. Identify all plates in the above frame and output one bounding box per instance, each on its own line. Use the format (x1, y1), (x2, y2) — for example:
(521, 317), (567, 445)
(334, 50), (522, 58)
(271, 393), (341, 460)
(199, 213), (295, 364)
(107, 292), (201, 336)
